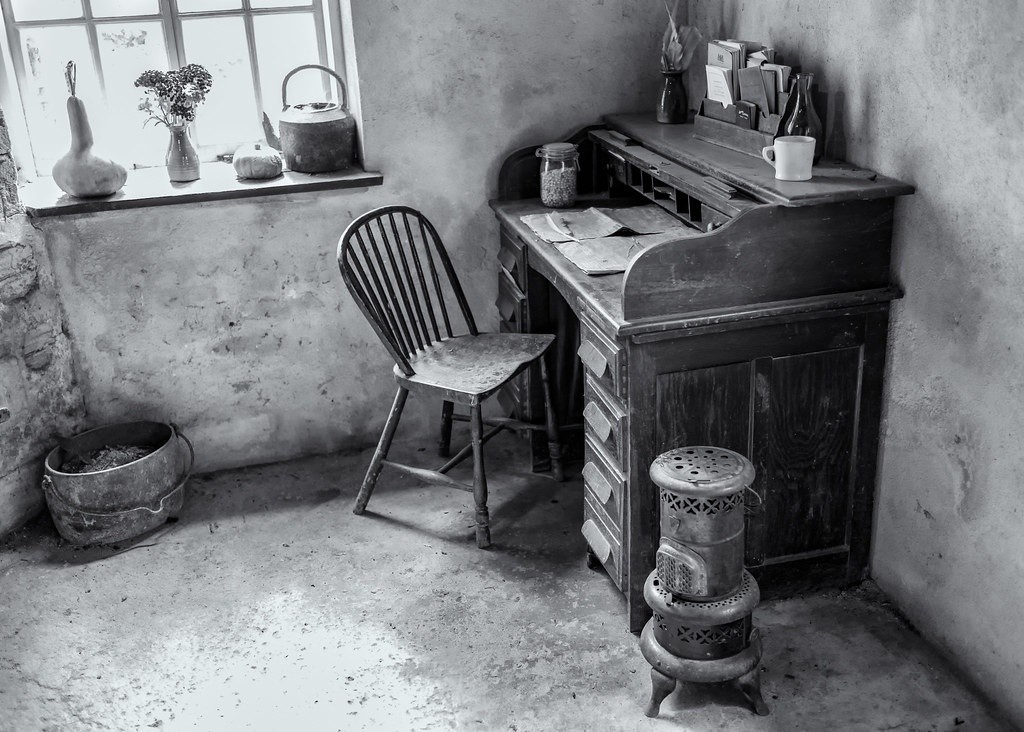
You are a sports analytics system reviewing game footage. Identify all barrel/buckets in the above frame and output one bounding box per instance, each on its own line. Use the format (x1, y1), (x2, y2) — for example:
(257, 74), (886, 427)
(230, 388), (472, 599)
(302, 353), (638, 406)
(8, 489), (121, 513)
(42, 420), (194, 545)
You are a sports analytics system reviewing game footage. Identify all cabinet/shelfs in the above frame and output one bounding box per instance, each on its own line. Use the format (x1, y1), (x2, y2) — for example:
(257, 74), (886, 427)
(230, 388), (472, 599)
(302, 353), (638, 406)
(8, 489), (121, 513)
(488, 107), (918, 634)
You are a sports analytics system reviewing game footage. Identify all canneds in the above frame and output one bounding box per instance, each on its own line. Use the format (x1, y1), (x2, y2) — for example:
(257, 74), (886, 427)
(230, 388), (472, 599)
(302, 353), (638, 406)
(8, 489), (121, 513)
(535, 142), (580, 208)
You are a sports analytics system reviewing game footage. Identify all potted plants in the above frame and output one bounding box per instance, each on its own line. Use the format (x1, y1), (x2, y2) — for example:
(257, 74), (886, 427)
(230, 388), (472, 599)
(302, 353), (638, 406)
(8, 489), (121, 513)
(656, 6), (701, 124)
(47, 56), (127, 199)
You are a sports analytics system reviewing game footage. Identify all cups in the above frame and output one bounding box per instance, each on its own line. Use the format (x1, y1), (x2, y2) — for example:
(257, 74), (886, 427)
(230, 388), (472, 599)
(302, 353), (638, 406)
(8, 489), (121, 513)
(762, 136), (816, 181)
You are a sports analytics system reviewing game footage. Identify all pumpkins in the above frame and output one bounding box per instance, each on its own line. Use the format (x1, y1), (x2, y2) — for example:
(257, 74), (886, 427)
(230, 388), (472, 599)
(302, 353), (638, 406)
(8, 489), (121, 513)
(51, 61), (127, 197)
(233, 143), (282, 179)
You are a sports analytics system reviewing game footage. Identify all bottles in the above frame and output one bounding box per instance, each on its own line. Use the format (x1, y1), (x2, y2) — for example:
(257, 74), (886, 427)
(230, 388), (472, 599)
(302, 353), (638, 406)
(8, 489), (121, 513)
(784, 72), (823, 166)
(657, 68), (689, 123)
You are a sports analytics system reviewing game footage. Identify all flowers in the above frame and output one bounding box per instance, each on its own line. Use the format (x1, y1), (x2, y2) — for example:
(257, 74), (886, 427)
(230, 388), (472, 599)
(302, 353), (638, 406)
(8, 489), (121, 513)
(135, 63), (212, 126)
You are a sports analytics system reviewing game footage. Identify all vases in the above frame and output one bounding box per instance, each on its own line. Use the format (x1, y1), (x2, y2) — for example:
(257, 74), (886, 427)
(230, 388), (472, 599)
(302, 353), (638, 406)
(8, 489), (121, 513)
(166, 123), (200, 183)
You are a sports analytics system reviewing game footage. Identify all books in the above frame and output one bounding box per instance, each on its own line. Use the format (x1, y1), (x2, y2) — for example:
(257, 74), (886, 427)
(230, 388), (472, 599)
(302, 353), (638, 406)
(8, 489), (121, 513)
(706, 38), (792, 118)
(738, 65), (778, 118)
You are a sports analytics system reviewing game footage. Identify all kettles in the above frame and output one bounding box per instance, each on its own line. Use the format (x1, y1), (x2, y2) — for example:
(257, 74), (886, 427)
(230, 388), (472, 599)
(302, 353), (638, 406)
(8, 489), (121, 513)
(260, 64), (357, 174)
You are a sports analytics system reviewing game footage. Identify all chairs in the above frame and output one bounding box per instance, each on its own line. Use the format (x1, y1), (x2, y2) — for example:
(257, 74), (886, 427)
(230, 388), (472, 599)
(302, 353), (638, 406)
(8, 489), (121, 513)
(335, 206), (563, 549)
